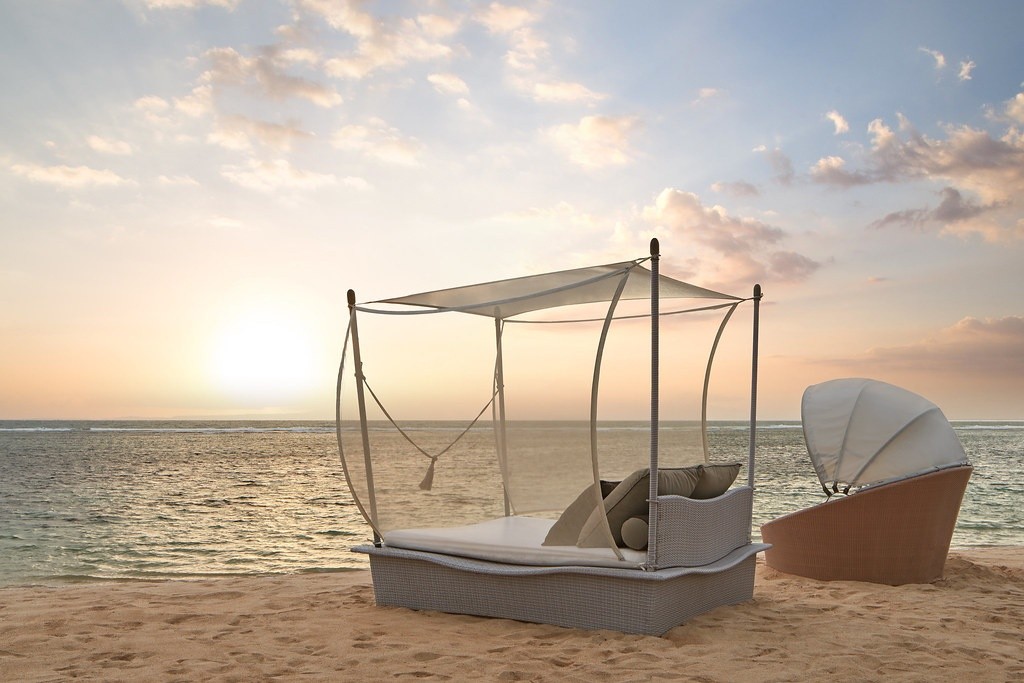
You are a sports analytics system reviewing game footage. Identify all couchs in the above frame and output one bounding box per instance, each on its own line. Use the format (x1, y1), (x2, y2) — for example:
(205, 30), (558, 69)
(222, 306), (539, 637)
(761, 459), (974, 588)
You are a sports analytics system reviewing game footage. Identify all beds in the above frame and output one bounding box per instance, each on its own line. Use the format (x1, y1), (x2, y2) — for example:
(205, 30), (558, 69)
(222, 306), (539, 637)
(351, 485), (771, 640)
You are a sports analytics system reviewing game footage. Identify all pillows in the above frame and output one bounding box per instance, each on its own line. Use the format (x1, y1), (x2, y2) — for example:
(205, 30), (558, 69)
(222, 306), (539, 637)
(576, 464), (703, 549)
(621, 514), (652, 552)
(690, 461), (743, 501)
(540, 479), (622, 546)
